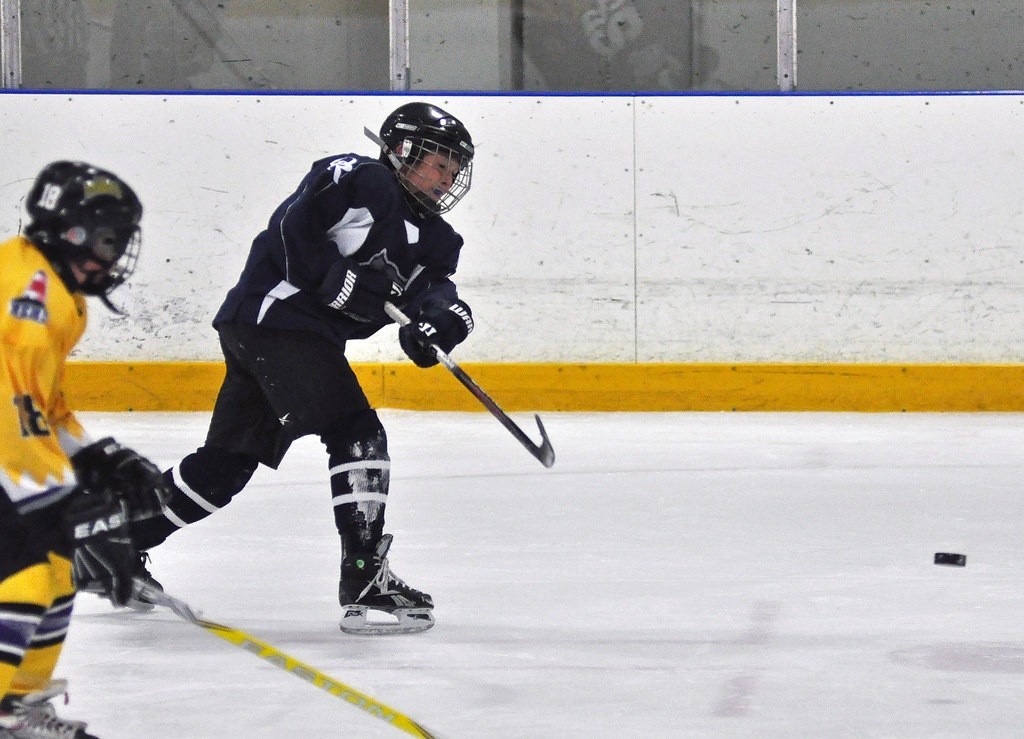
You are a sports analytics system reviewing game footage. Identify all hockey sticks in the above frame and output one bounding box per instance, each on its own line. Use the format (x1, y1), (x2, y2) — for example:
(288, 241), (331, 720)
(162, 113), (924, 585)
(383, 299), (555, 468)
(133, 577), (432, 739)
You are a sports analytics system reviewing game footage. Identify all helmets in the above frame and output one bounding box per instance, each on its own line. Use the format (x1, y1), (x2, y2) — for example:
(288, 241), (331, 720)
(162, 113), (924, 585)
(363, 103), (475, 221)
(24, 160), (142, 297)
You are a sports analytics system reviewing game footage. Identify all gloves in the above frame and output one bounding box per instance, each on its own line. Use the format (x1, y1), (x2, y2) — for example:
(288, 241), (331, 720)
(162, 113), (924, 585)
(69, 438), (172, 521)
(399, 299), (472, 369)
(312, 249), (402, 324)
(61, 489), (163, 612)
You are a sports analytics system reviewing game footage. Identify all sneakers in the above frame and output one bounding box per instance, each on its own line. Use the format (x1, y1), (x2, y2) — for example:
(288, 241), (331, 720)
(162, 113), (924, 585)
(100, 549), (157, 610)
(0, 678), (96, 739)
(338, 532), (434, 636)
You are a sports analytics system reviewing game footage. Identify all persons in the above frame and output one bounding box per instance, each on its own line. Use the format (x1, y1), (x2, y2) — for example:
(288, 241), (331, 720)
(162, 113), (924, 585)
(125, 102), (475, 637)
(0, 161), (173, 739)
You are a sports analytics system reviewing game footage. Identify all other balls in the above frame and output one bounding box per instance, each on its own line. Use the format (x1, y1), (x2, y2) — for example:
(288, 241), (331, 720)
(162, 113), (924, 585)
(933, 552), (967, 567)
